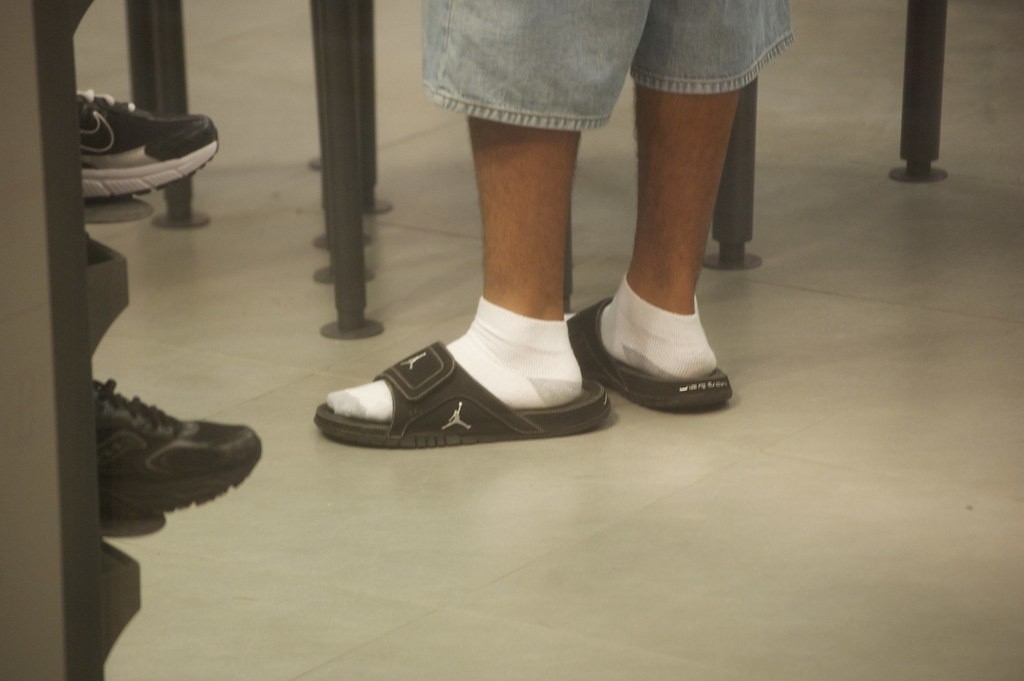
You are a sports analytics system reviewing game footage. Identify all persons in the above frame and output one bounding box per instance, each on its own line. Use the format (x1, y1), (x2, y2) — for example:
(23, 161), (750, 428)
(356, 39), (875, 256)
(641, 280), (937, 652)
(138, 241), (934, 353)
(312, 0), (796, 449)
(59, 0), (218, 199)
(93, 378), (262, 517)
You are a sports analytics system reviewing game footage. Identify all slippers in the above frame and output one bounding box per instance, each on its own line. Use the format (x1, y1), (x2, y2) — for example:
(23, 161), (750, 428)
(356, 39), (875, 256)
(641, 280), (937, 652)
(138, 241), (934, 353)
(314, 341), (611, 449)
(567, 298), (733, 411)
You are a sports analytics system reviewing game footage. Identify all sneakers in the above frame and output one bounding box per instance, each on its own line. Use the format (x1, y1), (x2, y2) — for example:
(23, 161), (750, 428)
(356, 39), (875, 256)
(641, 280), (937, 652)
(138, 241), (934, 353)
(93, 379), (261, 520)
(77, 89), (220, 199)
